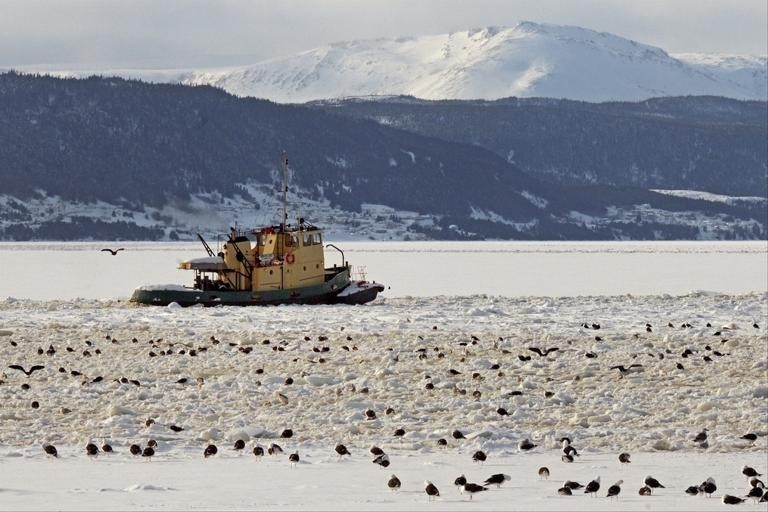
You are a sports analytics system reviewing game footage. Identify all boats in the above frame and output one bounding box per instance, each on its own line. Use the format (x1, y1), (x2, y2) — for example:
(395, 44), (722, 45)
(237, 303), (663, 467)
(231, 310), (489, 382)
(130, 151), (385, 305)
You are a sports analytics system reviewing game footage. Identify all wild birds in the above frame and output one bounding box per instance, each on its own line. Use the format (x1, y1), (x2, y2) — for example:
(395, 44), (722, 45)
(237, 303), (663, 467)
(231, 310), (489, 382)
(8, 322), (768, 505)
(101, 249), (124, 255)
